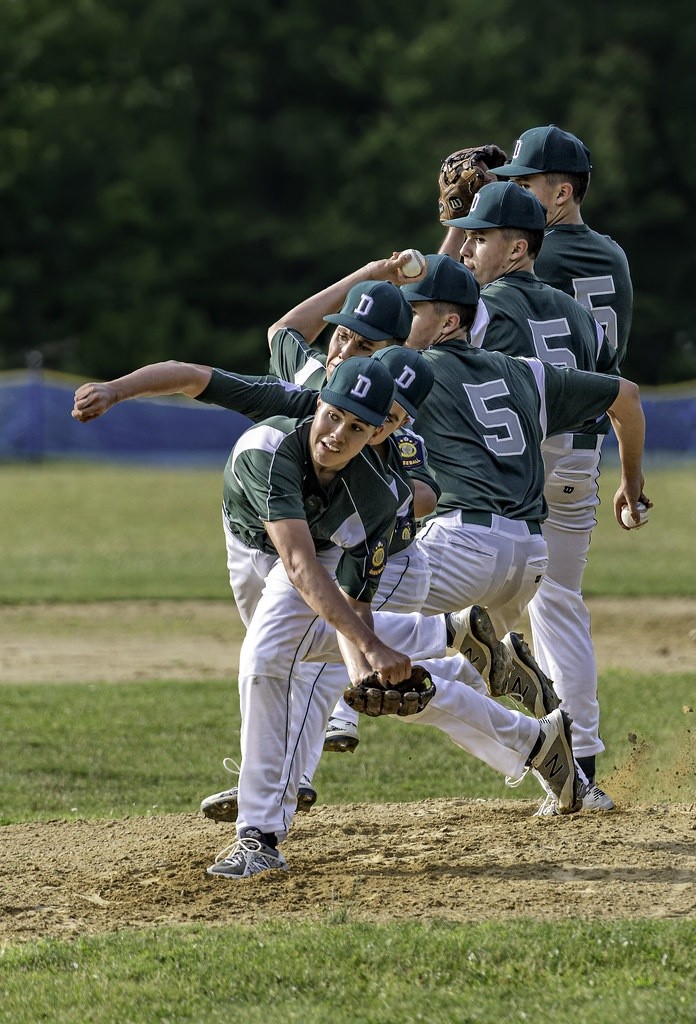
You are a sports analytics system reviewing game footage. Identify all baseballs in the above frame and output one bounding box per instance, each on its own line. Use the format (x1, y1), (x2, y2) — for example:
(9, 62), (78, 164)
(621, 502), (650, 531)
(397, 249), (426, 278)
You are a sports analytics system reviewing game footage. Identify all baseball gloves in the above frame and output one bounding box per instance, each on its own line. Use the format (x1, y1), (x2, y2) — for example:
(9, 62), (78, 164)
(343, 665), (436, 717)
(438, 144), (508, 222)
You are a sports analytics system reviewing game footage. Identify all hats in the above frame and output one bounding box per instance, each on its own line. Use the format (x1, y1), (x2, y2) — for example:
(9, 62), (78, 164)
(322, 279), (413, 343)
(320, 356), (399, 427)
(445, 180), (548, 230)
(400, 254), (480, 306)
(371, 345), (434, 419)
(486, 124), (591, 177)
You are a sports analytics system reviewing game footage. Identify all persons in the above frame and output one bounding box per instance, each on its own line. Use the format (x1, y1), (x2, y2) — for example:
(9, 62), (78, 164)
(71, 124), (654, 880)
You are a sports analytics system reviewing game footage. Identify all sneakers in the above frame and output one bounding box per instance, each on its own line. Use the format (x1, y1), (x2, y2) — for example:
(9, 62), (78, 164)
(199, 782), (318, 825)
(207, 827), (289, 878)
(531, 709), (579, 813)
(532, 785), (615, 818)
(499, 629), (564, 718)
(447, 604), (515, 698)
(323, 716), (360, 754)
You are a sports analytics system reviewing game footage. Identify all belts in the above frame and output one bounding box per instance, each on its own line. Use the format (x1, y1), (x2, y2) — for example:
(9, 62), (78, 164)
(462, 510), (543, 536)
(571, 435), (597, 450)
